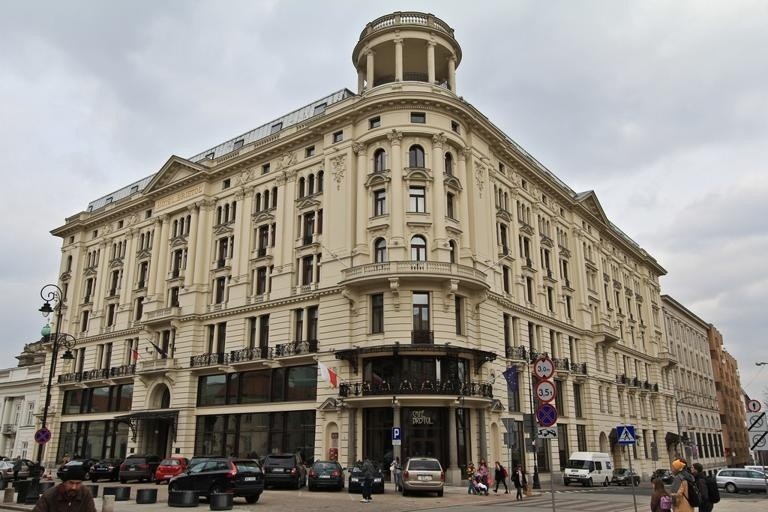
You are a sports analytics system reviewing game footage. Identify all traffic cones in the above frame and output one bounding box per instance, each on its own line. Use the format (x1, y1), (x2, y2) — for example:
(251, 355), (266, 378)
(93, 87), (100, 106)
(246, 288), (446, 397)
(47, 469), (53, 480)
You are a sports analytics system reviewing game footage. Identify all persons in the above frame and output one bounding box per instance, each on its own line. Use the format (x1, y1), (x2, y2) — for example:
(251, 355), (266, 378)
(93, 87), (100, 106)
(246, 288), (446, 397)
(13, 456), (22, 481)
(690, 463), (720, 512)
(360, 456), (375, 502)
(465, 458), (527, 501)
(650, 479), (671, 512)
(670, 458), (702, 512)
(32, 470), (96, 512)
(390, 457), (401, 491)
(62, 452), (70, 464)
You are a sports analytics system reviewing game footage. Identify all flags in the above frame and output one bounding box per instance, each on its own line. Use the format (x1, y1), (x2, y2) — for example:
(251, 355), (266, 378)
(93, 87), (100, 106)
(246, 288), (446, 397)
(502, 365), (517, 392)
(320, 363), (337, 386)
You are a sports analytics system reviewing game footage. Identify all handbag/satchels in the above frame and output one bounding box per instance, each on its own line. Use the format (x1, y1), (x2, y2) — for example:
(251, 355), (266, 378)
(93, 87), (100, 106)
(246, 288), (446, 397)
(659, 495), (672, 509)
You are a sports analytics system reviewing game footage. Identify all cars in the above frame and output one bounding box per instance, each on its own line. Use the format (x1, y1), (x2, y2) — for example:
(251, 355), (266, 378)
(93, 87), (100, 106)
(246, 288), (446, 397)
(650, 467), (672, 484)
(0, 460), (29, 481)
(19, 458), (46, 478)
(611, 466), (641, 487)
(307, 458), (348, 491)
(346, 464), (386, 494)
(56, 458), (97, 483)
(154, 456), (189, 485)
(89, 457), (123, 483)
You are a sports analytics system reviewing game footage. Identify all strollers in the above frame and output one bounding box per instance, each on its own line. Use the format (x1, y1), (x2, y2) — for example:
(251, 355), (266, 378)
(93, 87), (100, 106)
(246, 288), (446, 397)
(469, 476), (489, 496)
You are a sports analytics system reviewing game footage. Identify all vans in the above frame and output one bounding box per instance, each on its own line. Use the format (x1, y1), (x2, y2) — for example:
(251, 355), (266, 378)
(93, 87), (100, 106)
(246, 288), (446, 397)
(743, 465), (768, 474)
(715, 468), (768, 494)
(561, 450), (614, 487)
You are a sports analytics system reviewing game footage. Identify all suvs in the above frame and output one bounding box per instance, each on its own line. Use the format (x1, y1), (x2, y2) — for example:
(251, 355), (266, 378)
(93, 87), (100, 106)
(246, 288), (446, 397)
(166, 457), (267, 505)
(263, 452), (308, 490)
(186, 454), (228, 471)
(119, 453), (162, 485)
(394, 455), (446, 497)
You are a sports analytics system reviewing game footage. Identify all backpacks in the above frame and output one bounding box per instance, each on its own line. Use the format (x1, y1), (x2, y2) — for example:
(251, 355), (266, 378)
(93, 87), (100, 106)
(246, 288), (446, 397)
(682, 478), (703, 506)
(698, 476), (720, 503)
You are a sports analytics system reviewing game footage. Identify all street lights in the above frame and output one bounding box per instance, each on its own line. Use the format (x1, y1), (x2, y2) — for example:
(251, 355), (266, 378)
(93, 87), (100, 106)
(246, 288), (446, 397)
(675, 396), (696, 459)
(36, 283), (80, 464)
(754, 362), (768, 367)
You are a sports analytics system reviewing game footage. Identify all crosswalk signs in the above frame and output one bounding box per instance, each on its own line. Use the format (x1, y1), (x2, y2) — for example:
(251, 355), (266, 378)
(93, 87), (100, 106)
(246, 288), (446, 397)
(617, 426), (636, 445)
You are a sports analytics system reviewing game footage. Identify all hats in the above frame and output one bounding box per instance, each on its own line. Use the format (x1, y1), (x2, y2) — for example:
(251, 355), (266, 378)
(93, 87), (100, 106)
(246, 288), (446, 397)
(672, 459), (686, 471)
(57, 466), (92, 482)
